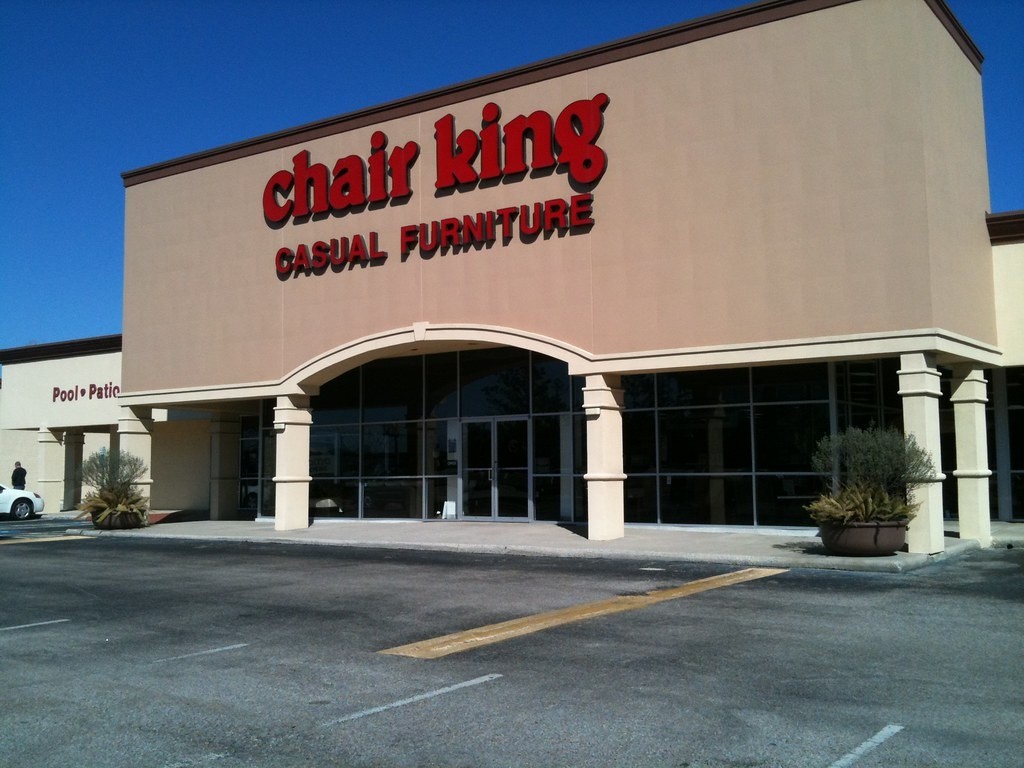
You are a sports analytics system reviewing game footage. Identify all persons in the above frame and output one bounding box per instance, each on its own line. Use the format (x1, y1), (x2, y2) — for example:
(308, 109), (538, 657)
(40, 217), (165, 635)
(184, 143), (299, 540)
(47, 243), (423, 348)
(12, 462), (27, 490)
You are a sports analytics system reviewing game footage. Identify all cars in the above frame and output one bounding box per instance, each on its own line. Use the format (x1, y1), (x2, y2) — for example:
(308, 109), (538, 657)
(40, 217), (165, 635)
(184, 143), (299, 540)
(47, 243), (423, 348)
(0, 483), (45, 521)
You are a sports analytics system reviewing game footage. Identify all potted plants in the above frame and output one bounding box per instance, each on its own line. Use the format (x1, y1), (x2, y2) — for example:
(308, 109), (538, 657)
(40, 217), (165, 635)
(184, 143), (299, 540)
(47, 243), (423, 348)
(72, 445), (149, 530)
(802, 427), (936, 557)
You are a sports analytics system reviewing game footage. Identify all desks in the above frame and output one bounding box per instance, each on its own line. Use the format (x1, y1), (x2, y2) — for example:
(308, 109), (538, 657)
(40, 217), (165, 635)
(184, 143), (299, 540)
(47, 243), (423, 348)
(346, 480), (417, 517)
